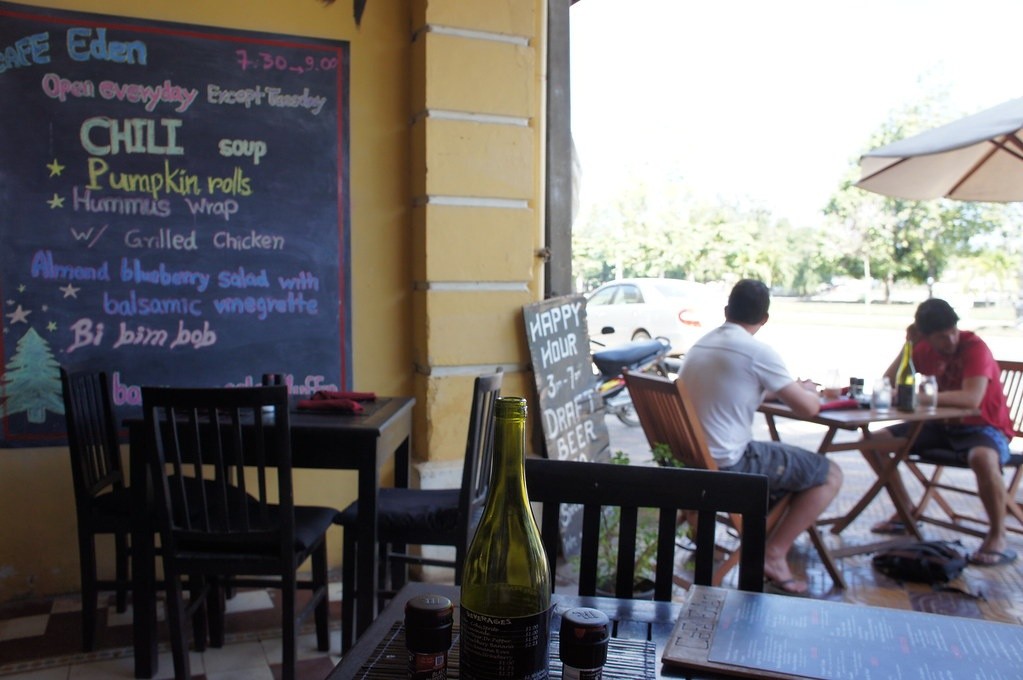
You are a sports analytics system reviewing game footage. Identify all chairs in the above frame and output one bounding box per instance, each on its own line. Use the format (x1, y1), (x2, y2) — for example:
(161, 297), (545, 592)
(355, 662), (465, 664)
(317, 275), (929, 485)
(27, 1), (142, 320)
(904, 359), (1023, 539)
(60, 354), (339, 680)
(525, 458), (770, 602)
(623, 368), (794, 589)
(336, 365), (504, 656)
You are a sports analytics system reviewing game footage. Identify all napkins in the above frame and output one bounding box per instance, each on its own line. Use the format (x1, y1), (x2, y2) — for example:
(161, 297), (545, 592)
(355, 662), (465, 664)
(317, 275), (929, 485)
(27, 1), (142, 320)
(820, 399), (860, 410)
(312, 390), (377, 402)
(296, 398), (361, 411)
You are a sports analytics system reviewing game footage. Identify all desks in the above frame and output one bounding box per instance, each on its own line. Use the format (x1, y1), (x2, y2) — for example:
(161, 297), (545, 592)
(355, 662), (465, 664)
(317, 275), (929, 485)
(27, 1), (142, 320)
(757, 400), (983, 587)
(323, 581), (750, 680)
(123, 396), (416, 680)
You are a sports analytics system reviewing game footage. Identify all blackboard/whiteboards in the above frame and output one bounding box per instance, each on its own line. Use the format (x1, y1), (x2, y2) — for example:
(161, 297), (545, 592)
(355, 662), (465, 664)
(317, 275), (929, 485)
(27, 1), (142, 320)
(0, 0), (353, 448)
(521, 296), (617, 562)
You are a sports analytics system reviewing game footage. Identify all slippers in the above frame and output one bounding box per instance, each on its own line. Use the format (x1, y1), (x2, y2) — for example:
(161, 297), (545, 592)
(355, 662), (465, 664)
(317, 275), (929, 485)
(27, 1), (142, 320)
(871, 517), (923, 535)
(967, 548), (1017, 566)
(763, 573), (811, 596)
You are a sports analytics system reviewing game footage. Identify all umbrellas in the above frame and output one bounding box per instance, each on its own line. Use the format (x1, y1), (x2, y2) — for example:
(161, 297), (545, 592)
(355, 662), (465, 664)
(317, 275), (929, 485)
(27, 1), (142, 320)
(851, 97), (1023, 202)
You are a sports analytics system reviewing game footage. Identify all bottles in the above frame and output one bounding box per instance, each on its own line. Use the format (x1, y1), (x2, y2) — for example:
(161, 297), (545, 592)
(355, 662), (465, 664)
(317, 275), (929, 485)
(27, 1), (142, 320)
(460, 396), (552, 680)
(848, 377), (864, 396)
(260, 374), (284, 412)
(559, 608), (609, 680)
(404, 597), (454, 680)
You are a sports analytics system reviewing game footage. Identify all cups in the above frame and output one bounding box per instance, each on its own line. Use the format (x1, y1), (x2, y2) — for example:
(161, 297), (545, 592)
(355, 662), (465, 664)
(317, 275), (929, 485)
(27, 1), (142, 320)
(912, 375), (938, 414)
(898, 384), (913, 411)
(871, 377), (892, 414)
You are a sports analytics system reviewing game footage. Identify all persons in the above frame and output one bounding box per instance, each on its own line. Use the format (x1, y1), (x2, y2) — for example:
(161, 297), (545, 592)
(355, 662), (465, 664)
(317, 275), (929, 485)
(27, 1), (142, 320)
(859, 298), (1017, 566)
(675, 278), (843, 597)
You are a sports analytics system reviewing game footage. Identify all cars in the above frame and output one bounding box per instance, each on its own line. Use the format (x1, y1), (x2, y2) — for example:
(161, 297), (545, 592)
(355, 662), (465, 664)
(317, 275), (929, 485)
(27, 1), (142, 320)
(584, 277), (727, 357)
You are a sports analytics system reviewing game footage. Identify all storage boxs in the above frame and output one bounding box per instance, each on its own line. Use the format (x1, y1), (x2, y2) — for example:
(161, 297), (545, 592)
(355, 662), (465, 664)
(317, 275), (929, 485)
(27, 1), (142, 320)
(569, 442), (697, 600)
